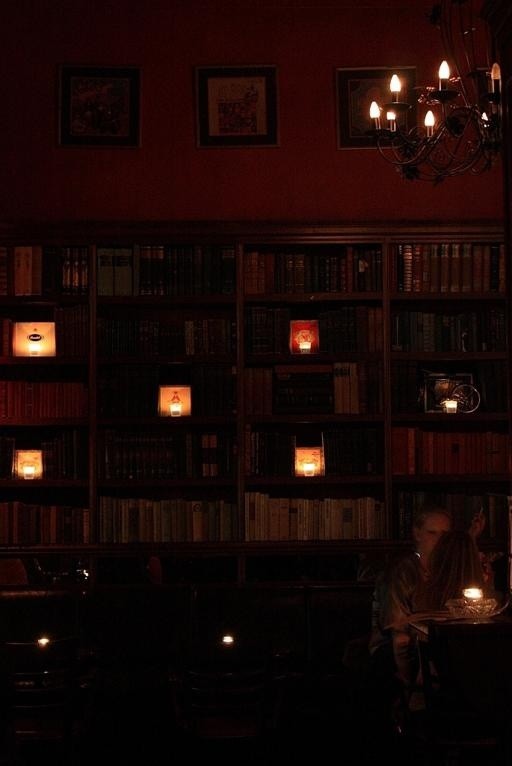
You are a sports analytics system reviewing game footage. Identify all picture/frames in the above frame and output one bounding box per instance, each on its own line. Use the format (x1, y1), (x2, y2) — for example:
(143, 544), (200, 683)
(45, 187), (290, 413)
(194, 63), (281, 152)
(55, 62), (145, 153)
(424, 370), (474, 416)
(334, 63), (419, 153)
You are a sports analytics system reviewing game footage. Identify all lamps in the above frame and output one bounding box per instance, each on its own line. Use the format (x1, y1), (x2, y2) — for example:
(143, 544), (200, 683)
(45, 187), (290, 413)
(369, 2), (505, 188)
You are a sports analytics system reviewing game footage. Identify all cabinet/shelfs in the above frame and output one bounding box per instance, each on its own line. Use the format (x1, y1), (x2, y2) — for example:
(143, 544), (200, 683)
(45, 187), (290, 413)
(3, 215), (500, 598)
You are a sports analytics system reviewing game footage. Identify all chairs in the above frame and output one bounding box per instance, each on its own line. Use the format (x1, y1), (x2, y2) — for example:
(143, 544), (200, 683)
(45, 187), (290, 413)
(426, 623), (510, 764)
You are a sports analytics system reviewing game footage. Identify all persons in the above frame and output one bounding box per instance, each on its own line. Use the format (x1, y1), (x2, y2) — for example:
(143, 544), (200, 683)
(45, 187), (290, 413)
(370, 503), (506, 740)
